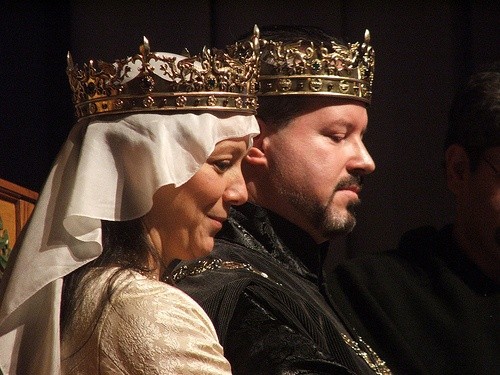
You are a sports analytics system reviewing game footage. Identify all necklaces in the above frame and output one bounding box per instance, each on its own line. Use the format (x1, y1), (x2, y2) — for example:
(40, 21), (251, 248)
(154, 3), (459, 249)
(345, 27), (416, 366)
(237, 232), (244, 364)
(129, 261), (160, 279)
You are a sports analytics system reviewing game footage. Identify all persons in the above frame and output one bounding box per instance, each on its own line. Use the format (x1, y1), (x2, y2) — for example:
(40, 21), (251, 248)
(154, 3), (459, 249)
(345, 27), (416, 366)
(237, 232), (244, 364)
(158, 27), (402, 375)
(2, 26), (264, 375)
(345, 73), (500, 375)
